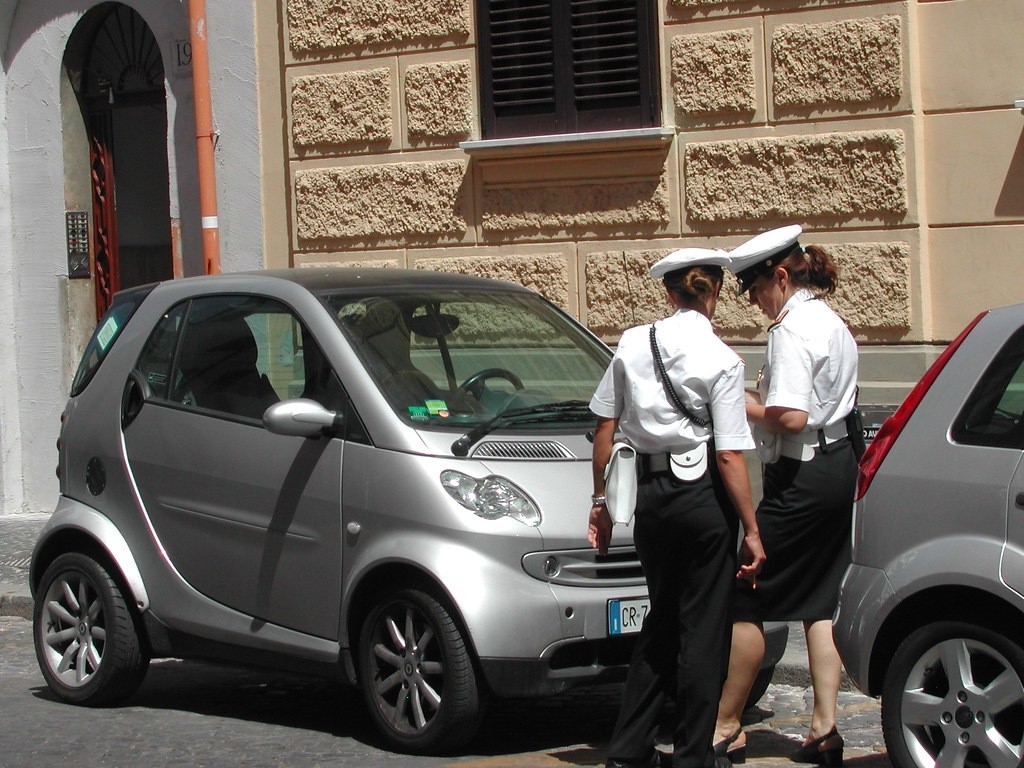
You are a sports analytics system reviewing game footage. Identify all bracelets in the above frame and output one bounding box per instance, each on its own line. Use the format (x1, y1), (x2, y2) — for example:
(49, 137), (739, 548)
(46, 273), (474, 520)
(592, 495), (605, 505)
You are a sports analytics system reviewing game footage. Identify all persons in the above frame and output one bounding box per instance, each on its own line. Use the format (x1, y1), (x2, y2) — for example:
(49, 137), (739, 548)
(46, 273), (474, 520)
(713, 224), (861, 765)
(588, 246), (766, 768)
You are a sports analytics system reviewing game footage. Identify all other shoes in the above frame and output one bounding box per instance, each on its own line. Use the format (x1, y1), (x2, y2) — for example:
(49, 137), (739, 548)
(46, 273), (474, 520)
(707, 757), (733, 768)
(608, 751), (673, 768)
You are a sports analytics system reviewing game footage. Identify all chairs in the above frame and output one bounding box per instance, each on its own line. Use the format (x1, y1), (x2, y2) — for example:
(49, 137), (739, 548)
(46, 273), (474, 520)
(174, 303), (281, 419)
(316, 296), (446, 434)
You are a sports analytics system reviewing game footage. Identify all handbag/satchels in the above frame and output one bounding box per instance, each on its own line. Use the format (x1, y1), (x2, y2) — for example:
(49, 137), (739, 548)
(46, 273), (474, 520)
(604, 442), (637, 526)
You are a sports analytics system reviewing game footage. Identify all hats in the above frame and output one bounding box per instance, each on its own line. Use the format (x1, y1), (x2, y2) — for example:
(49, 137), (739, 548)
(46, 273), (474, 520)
(728, 224), (802, 296)
(649, 247), (730, 280)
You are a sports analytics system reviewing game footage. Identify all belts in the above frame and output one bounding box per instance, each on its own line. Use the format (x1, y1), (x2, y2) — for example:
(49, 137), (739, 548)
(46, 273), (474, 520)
(779, 420), (852, 462)
(639, 451), (670, 473)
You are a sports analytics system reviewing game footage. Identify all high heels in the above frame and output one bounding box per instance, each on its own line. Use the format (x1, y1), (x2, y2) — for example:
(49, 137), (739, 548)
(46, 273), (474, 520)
(790, 730), (845, 767)
(713, 730), (748, 764)
